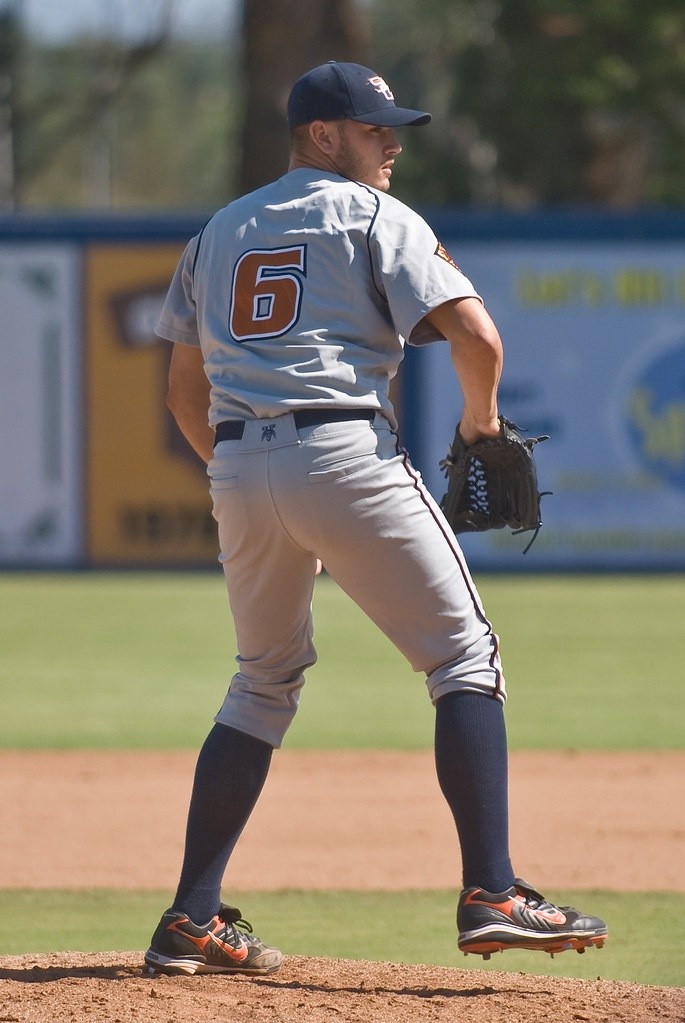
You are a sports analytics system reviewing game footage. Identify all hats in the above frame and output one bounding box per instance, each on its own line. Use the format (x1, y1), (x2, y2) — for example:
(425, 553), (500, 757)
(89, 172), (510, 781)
(287, 61), (433, 128)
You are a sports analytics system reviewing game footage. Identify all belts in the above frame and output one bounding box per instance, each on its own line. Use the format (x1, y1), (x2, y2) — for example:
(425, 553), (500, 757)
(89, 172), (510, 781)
(211, 408), (377, 448)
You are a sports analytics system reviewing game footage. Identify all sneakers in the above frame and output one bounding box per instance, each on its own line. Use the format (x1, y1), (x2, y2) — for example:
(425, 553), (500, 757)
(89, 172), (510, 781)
(143, 903), (283, 976)
(456, 879), (609, 960)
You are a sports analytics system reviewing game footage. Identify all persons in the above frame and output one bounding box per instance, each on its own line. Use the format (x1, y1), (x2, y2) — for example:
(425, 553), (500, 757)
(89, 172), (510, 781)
(145, 60), (607, 973)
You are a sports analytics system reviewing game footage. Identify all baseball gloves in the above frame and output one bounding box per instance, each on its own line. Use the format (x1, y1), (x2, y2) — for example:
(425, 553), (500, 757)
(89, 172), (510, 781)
(438, 412), (555, 558)
